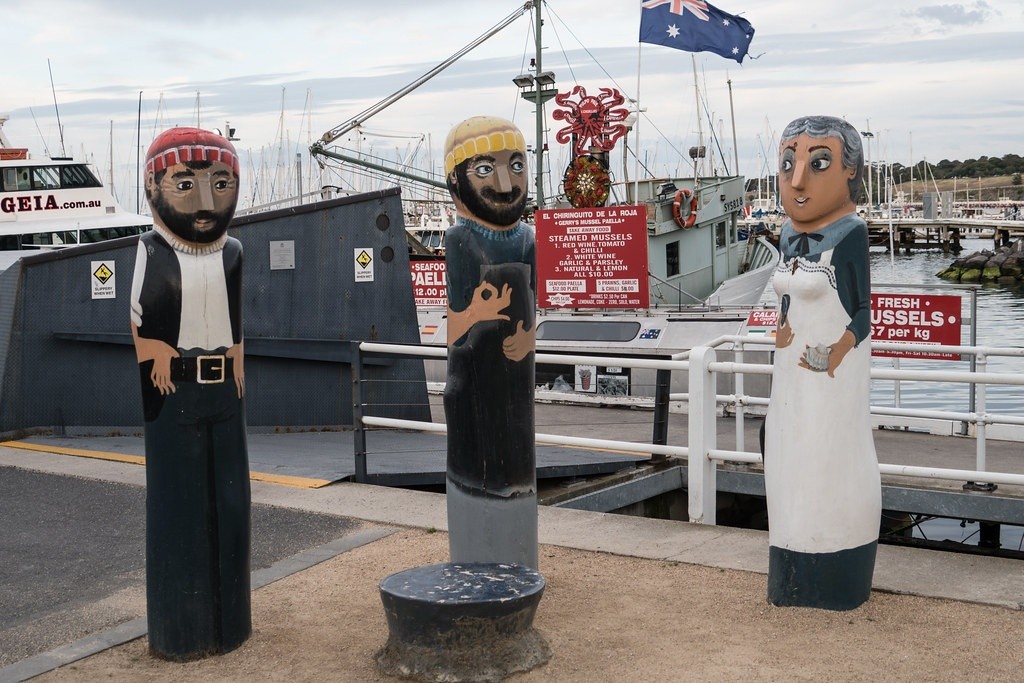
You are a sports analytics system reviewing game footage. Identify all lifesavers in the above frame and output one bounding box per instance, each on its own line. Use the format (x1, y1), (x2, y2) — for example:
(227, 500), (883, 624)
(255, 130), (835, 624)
(673, 189), (697, 230)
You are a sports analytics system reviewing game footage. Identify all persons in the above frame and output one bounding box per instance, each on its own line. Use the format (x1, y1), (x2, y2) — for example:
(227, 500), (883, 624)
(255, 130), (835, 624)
(1000, 203), (1018, 220)
(130, 128), (251, 664)
(442, 118), (540, 575)
(760, 116), (882, 612)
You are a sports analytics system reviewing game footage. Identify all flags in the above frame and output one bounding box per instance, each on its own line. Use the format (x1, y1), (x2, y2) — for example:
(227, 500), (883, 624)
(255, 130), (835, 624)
(639, 0), (756, 63)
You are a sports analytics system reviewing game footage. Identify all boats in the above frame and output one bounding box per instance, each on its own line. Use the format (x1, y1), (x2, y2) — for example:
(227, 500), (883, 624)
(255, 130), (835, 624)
(1, 0), (1024, 436)
